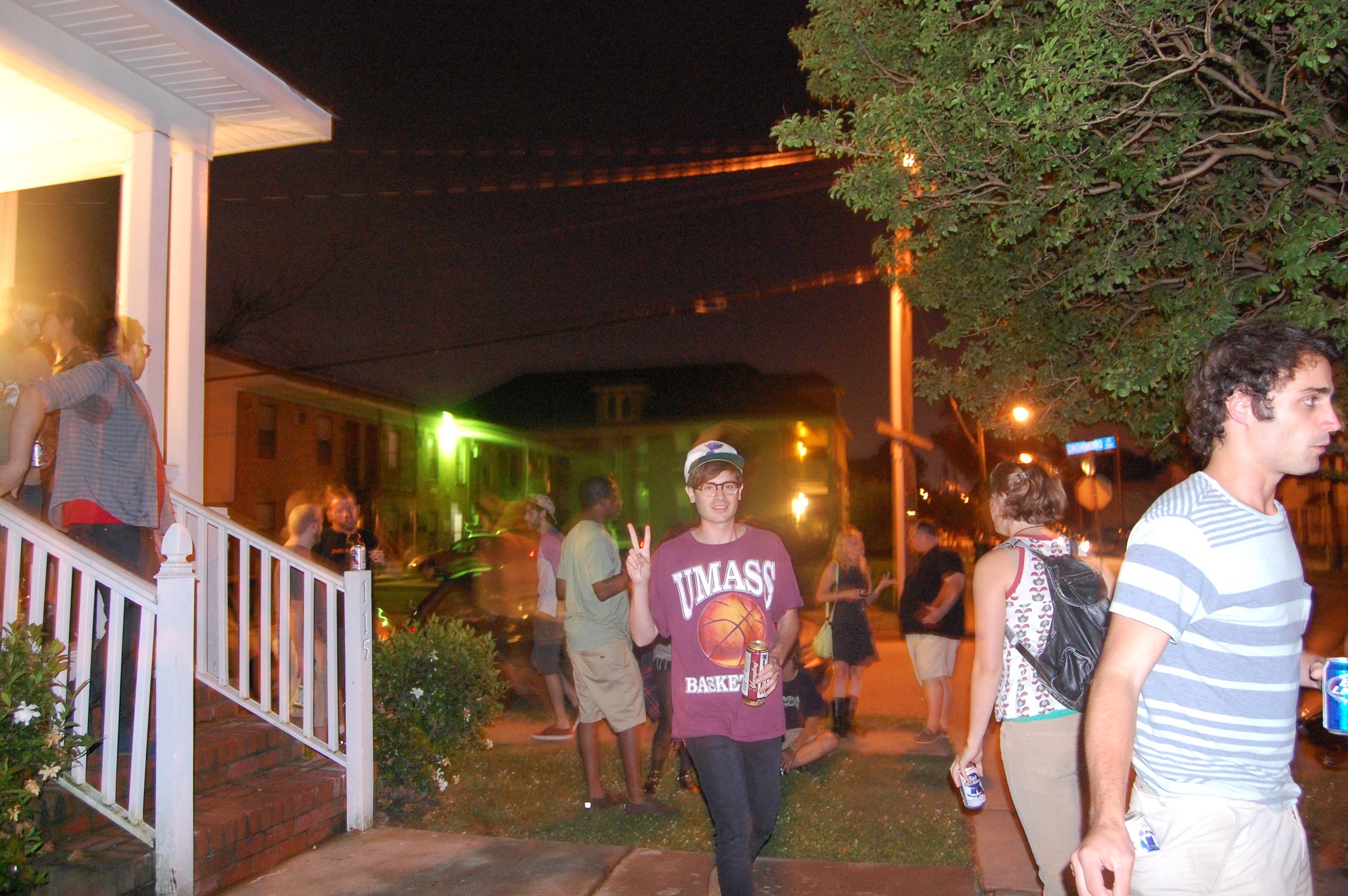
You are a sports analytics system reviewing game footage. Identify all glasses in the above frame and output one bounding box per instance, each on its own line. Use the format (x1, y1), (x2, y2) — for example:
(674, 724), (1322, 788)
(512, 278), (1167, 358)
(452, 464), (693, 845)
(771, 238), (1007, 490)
(132, 341), (153, 358)
(691, 481), (742, 497)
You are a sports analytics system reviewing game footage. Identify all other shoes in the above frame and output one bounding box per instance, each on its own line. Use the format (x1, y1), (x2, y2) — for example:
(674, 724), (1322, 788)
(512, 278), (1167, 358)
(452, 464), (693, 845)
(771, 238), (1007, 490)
(584, 788), (626, 812)
(1323, 740), (1348, 764)
(625, 796), (680, 818)
(677, 771), (701, 793)
(96, 730), (157, 757)
(707, 866), (721, 896)
(915, 727), (944, 743)
(642, 769), (661, 793)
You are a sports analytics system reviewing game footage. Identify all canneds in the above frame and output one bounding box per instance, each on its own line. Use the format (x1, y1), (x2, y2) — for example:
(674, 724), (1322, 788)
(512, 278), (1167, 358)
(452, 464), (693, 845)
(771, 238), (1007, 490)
(741, 641), (769, 707)
(350, 545), (366, 570)
(1124, 810), (1162, 852)
(958, 763), (986, 809)
(31, 440), (47, 468)
(1321, 657), (1348, 735)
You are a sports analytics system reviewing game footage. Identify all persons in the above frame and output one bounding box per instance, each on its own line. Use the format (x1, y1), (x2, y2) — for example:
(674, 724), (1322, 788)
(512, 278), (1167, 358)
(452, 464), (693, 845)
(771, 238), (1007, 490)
(643, 636), (701, 797)
(812, 528), (900, 738)
(951, 460), (1118, 896)
(524, 494), (581, 740)
(624, 441), (802, 896)
(274, 502), (329, 760)
(311, 491), (387, 743)
(898, 520), (967, 746)
(1068, 323), (1348, 896)
(555, 472), (681, 818)
(0, 279), (175, 757)
(774, 638), (838, 773)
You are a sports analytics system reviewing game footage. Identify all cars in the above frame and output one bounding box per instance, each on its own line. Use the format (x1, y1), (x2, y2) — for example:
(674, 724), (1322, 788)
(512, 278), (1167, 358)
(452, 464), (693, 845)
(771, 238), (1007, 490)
(399, 568), (541, 649)
(407, 533), (539, 581)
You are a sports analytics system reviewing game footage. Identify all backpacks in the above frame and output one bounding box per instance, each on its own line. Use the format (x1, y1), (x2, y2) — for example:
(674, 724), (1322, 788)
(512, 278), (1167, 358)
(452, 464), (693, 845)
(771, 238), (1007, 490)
(988, 539), (1111, 711)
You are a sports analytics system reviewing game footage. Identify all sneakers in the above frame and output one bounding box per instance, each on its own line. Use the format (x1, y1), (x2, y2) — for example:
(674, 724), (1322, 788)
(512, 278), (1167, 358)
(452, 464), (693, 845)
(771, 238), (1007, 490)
(571, 704), (580, 732)
(531, 725), (574, 740)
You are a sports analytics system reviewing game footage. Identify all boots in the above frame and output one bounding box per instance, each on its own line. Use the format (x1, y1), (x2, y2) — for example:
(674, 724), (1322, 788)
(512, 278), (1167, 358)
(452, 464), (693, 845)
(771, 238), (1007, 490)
(829, 699), (848, 738)
(843, 694), (861, 734)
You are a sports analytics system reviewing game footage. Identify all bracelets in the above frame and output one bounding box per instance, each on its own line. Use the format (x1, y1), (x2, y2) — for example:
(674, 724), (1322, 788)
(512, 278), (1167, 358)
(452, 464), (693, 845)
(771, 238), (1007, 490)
(789, 742), (800, 749)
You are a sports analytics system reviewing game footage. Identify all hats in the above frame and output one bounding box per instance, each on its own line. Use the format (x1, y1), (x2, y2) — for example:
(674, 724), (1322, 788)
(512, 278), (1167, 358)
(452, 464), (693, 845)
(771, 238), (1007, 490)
(533, 494), (558, 525)
(684, 440), (744, 483)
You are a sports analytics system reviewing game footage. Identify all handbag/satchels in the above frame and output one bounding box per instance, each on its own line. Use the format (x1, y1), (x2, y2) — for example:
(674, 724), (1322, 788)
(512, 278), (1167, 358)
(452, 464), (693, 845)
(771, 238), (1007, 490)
(813, 621), (833, 659)
